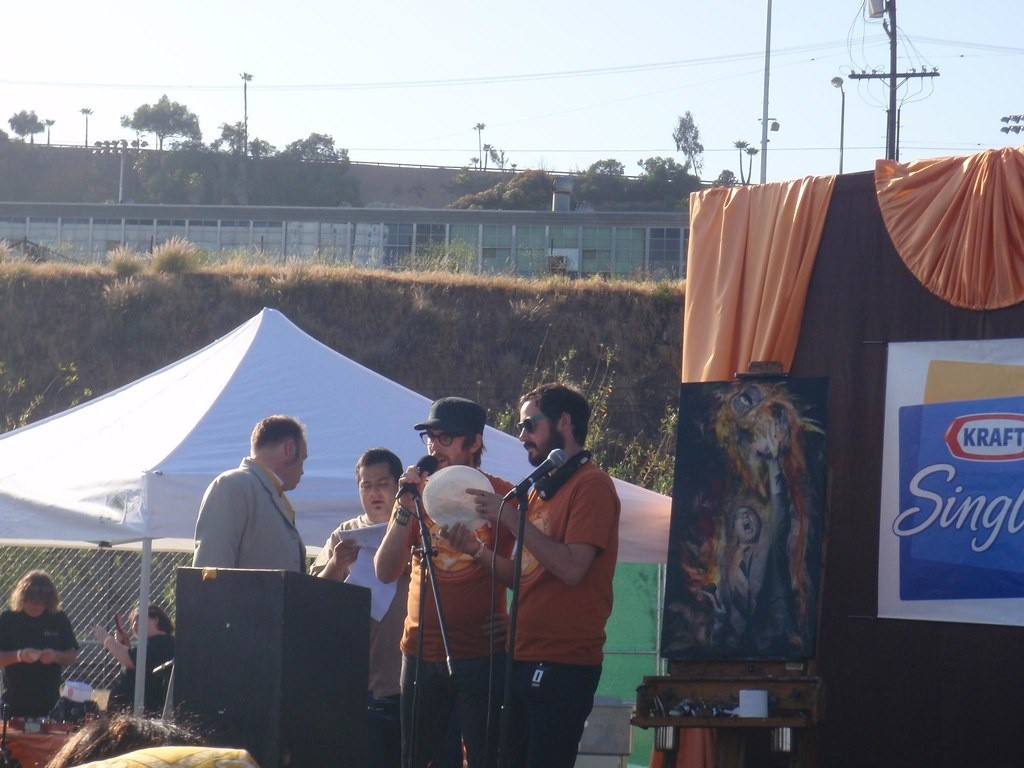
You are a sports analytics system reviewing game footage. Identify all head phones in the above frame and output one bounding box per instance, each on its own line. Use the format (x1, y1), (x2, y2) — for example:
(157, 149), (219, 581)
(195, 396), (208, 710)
(534, 451), (592, 500)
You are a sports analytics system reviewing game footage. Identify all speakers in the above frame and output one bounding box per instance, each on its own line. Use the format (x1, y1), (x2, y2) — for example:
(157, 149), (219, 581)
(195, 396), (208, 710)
(172, 565), (372, 768)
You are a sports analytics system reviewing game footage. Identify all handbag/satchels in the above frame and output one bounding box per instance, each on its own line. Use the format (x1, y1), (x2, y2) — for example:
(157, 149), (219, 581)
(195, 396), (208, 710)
(49, 697), (95, 724)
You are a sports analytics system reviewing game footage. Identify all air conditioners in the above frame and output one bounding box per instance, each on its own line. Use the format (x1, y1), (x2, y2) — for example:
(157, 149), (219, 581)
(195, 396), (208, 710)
(546, 255), (567, 270)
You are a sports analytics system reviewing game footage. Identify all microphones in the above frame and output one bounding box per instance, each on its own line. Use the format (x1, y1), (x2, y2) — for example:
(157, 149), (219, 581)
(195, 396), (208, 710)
(394, 455), (439, 498)
(501, 449), (567, 501)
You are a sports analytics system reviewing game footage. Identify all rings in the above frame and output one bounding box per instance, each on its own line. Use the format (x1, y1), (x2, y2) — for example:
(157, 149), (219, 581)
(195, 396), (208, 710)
(481, 505), (484, 512)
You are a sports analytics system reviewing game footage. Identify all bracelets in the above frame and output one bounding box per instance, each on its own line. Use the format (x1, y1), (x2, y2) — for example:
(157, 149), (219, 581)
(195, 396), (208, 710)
(17, 649), (22, 662)
(395, 510), (410, 525)
(474, 542), (483, 559)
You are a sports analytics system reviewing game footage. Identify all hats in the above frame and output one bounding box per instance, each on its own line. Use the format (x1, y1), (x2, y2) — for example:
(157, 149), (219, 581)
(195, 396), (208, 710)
(414, 397), (485, 435)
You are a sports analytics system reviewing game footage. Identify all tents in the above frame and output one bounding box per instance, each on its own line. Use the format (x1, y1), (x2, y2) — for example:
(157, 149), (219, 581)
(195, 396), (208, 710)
(0, 306), (671, 738)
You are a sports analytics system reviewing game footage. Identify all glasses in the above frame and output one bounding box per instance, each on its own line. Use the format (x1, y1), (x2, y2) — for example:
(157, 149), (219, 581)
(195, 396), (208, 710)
(518, 415), (548, 434)
(420, 431), (473, 447)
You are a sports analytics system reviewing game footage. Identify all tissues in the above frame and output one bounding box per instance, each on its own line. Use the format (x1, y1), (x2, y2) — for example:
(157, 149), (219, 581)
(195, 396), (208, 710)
(738, 689), (768, 718)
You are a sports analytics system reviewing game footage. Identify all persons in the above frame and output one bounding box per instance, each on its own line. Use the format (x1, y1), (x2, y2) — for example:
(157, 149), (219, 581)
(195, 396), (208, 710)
(191, 415), (308, 572)
(94, 605), (174, 709)
(308, 449), (413, 702)
(45, 712), (208, 768)
(439, 382), (621, 768)
(0, 572), (79, 721)
(373, 397), (528, 768)
